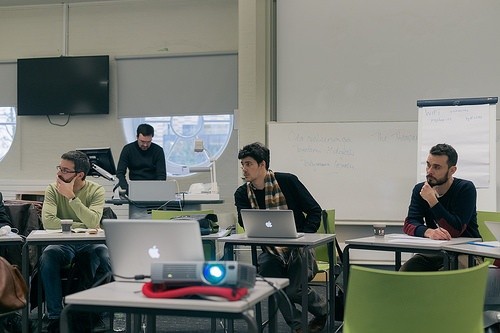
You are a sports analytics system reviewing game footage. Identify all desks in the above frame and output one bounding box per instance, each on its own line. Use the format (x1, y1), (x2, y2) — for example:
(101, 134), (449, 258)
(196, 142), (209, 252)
(218, 229), (336, 333)
(342, 233), (482, 297)
(441, 241), (500, 272)
(21, 228), (229, 332)
(58, 276), (290, 332)
(0, 232), (22, 261)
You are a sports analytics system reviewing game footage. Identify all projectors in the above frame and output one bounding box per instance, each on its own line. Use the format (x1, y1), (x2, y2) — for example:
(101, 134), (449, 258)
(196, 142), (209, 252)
(151, 261), (256, 289)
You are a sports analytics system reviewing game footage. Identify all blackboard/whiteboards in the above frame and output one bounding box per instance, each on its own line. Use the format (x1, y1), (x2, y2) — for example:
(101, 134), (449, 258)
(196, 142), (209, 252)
(266, 120), (419, 225)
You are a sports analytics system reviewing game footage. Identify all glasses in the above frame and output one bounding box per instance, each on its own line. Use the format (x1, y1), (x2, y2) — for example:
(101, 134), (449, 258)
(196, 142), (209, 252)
(57, 166), (81, 174)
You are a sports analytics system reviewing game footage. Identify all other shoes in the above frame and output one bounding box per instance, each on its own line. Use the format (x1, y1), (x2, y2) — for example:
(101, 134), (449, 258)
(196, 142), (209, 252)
(47, 319), (60, 333)
(93, 311), (107, 332)
(292, 328), (301, 333)
(309, 315), (326, 333)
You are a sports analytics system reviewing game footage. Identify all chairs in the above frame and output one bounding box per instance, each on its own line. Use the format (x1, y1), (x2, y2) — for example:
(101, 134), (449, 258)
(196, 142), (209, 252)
(0, 200), (500, 333)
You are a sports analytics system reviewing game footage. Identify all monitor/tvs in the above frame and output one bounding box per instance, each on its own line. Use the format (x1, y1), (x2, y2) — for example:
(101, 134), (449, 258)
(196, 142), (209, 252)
(17, 55), (109, 115)
(75, 147), (117, 177)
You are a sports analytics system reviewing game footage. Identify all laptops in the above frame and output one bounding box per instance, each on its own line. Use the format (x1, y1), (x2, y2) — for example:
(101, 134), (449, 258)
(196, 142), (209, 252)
(103, 219), (205, 283)
(240, 208), (305, 238)
(485, 221), (500, 241)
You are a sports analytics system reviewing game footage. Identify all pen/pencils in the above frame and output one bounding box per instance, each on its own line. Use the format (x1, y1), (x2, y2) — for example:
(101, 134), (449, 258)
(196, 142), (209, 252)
(433, 220), (439, 227)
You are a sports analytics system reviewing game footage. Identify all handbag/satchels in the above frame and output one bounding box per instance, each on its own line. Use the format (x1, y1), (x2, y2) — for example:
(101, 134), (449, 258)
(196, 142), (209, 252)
(0, 257), (27, 309)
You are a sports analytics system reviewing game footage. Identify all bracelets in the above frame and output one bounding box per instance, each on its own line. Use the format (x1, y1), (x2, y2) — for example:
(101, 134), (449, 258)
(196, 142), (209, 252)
(69, 196), (77, 203)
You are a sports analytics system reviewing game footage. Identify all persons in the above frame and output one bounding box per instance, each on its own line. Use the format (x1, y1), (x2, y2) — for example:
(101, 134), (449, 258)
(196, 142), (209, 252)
(399, 143), (483, 273)
(116, 124), (167, 219)
(0, 192), (23, 333)
(38, 150), (111, 333)
(234, 142), (331, 333)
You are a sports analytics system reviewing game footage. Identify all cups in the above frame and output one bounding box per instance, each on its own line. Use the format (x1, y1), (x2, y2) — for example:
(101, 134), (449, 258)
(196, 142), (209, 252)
(60, 220), (73, 235)
(118, 189), (126, 200)
(373, 223), (386, 239)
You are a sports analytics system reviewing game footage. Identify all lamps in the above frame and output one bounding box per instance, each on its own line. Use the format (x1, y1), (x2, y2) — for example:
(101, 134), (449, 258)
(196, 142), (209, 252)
(188, 140), (218, 193)
